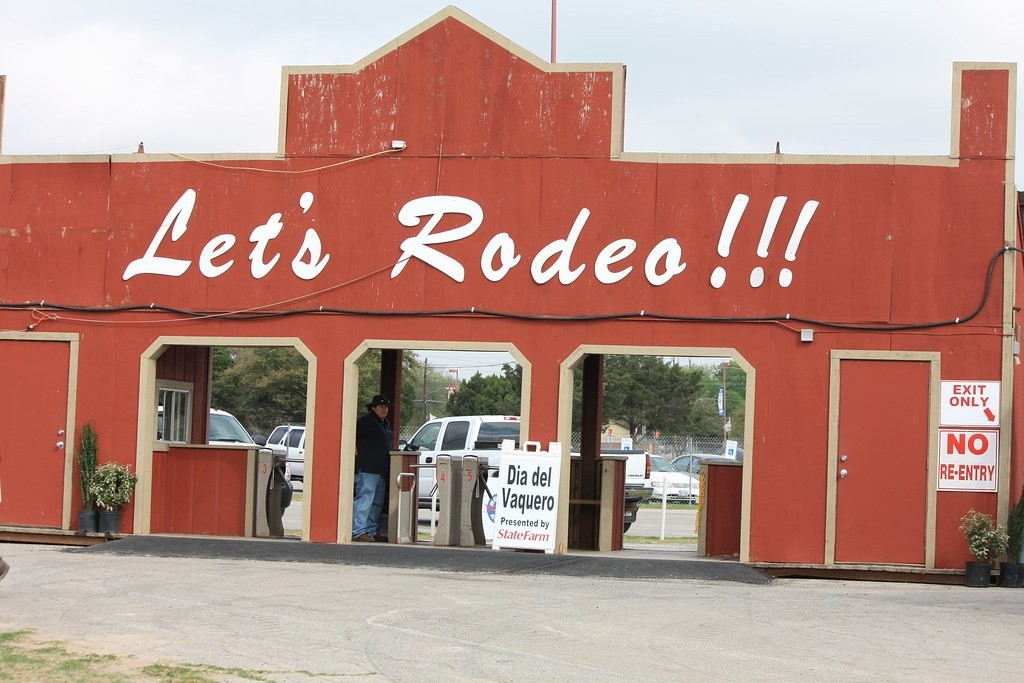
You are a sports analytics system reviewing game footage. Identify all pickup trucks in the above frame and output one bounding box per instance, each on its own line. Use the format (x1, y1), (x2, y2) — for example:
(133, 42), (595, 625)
(399, 414), (651, 532)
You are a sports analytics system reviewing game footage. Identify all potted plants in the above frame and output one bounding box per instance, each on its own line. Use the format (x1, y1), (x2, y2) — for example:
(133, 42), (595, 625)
(958, 486), (1024, 587)
(74, 420), (137, 534)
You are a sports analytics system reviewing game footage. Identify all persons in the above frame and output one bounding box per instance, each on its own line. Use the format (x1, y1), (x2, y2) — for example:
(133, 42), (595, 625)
(351, 394), (393, 541)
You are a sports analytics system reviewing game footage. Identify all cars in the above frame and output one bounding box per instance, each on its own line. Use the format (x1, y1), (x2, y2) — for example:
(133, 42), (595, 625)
(648, 454), (734, 504)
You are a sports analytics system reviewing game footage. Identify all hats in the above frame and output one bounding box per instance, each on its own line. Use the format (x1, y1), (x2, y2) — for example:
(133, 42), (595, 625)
(372, 395), (388, 407)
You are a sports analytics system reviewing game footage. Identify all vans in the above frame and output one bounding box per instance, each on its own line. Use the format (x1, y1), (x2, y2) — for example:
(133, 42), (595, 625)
(264, 425), (305, 481)
(156, 404), (293, 517)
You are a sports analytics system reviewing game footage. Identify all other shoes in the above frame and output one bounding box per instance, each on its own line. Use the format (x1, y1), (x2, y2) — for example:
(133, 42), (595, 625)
(352, 534), (388, 542)
